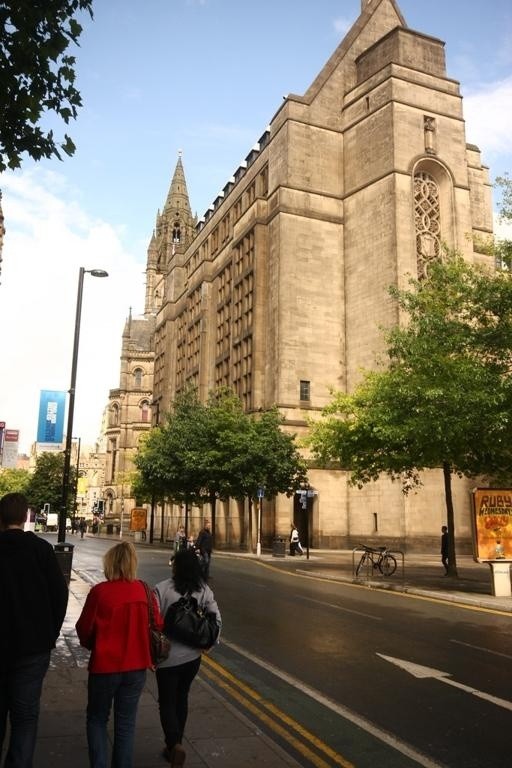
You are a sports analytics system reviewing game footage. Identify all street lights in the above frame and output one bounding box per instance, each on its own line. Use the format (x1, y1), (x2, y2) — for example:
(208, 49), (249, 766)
(71, 437), (81, 534)
(299, 477), (312, 560)
(149, 402), (159, 544)
(57, 266), (110, 580)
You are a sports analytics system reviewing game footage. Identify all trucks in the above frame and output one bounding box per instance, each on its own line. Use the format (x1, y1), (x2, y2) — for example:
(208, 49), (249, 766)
(43, 514), (72, 533)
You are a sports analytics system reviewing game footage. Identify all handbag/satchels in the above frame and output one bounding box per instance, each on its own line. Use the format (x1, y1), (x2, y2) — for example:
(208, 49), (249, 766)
(163, 589), (219, 649)
(150, 630), (170, 665)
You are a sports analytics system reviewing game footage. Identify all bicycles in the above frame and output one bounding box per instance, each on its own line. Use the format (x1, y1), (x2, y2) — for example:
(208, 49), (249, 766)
(356, 543), (397, 576)
(132, 531), (146, 542)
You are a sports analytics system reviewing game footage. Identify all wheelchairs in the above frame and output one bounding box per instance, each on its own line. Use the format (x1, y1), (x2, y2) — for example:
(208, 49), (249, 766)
(176, 540), (204, 566)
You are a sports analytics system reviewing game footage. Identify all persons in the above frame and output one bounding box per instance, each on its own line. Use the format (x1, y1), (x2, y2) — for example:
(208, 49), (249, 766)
(0, 492), (69, 768)
(71, 517), (99, 538)
(288, 523), (303, 556)
(441, 526), (449, 576)
(154, 548), (223, 767)
(76, 540), (164, 768)
(168, 520), (213, 579)
